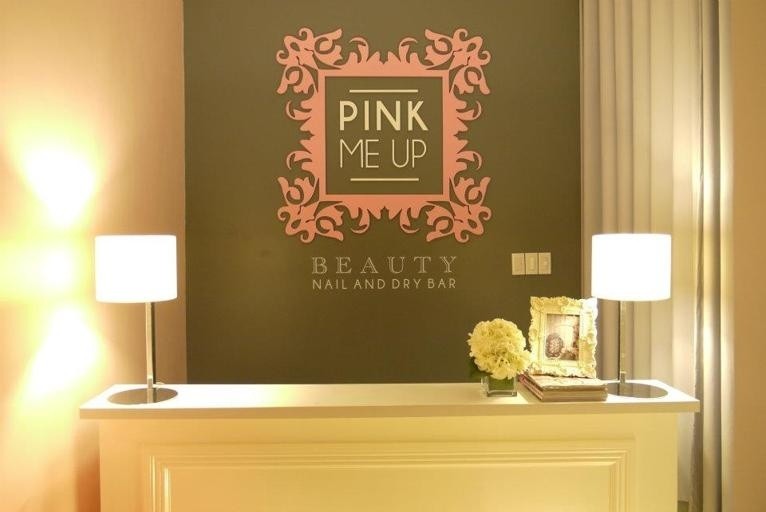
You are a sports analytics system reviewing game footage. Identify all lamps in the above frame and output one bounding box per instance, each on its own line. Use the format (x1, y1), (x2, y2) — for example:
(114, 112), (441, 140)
(93, 236), (179, 407)
(590, 234), (672, 401)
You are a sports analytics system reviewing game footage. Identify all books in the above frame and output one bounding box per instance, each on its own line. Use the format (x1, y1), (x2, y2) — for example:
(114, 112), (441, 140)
(520, 374), (609, 402)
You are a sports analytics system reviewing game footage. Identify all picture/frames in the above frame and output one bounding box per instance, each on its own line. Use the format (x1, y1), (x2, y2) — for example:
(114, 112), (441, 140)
(527, 294), (598, 378)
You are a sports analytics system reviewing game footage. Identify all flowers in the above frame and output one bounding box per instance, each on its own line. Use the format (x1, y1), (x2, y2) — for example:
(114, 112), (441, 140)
(467, 319), (530, 381)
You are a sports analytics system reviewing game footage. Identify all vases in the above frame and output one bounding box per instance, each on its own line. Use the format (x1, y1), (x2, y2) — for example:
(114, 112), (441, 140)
(485, 374), (515, 396)
